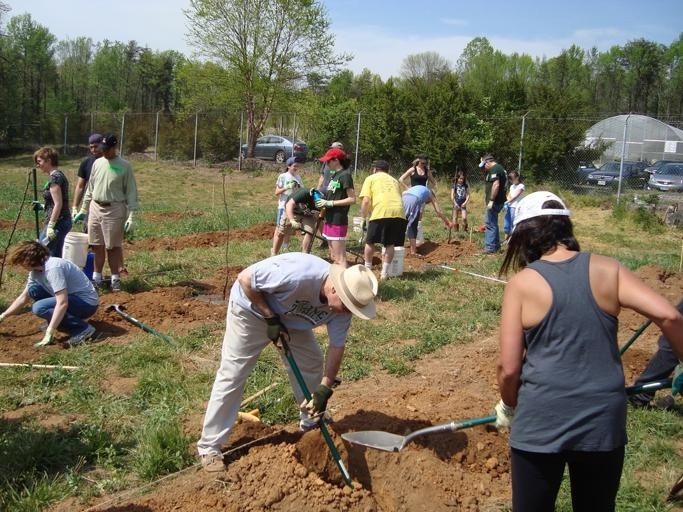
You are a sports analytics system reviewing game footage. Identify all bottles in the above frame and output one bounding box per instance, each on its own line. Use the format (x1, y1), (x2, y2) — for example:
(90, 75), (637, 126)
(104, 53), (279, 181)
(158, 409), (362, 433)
(312, 190), (322, 210)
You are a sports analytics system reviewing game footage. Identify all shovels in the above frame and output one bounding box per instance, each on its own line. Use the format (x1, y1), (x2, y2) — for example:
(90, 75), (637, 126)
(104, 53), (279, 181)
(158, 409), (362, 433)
(340, 378), (673, 453)
(104, 304), (173, 344)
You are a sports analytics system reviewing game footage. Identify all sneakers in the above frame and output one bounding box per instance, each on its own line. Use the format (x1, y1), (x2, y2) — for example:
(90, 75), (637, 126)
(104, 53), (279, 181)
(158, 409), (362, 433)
(110, 279), (121, 291)
(68, 324), (95, 345)
(92, 280), (104, 287)
(201, 454), (226, 472)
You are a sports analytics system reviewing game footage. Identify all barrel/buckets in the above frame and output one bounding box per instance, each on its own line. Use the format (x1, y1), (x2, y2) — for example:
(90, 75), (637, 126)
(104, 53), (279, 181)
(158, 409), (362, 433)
(62, 232), (96, 281)
(352, 217), (368, 233)
(381, 245), (405, 278)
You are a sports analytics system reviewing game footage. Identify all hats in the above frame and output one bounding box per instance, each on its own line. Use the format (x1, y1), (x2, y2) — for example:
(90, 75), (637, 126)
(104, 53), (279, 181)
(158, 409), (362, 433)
(479, 154), (494, 167)
(371, 160), (389, 170)
(89, 134), (103, 143)
(319, 148), (344, 161)
(330, 142), (344, 149)
(328, 263), (378, 321)
(99, 136), (117, 148)
(506, 191), (570, 243)
(285, 157), (299, 170)
(419, 155), (428, 162)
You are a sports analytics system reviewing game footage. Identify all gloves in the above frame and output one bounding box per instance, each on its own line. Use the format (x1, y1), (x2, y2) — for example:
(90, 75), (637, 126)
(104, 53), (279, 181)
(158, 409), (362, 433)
(47, 221), (56, 241)
(34, 327), (56, 346)
(672, 360), (683, 399)
(264, 314), (290, 350)
(300, 384), (333, 423)
(361, 218), (367, 231)
(32, 202), (44, 210)
(72, 207), (78, 219)
(316, 199), (333, 208)
(493, 399), (514, 437)
(286, 181), (296, 188)
(74, 208), (87, 225)
(124, 211), (133, 232)
(290, 219), (300, 230)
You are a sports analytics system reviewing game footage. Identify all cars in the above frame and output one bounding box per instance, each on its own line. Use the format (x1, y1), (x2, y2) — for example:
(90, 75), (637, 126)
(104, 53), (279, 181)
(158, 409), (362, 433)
(241, 134), (307, 164)
(644, 160), (683, 176)
(576, 159), (598, 185)
(648, 163), (682, 193)
(586, 161), (650, 189)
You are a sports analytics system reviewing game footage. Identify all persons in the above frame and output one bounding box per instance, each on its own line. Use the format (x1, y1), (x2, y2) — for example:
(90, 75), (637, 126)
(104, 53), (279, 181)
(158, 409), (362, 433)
(626, 298), (683, 409)
(32, 144), (73, 258)
(270, 139), (526, 281)
(74, 132), (138, 293)
(195, 250), (381, 476)
(70, 133), (129, 278)
(492, 189), (683, 512)
(0, 240), (101, 349)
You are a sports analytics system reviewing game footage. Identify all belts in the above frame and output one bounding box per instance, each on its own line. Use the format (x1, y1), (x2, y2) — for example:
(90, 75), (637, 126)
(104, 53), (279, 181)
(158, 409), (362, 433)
(95, 200), (110, 206)
